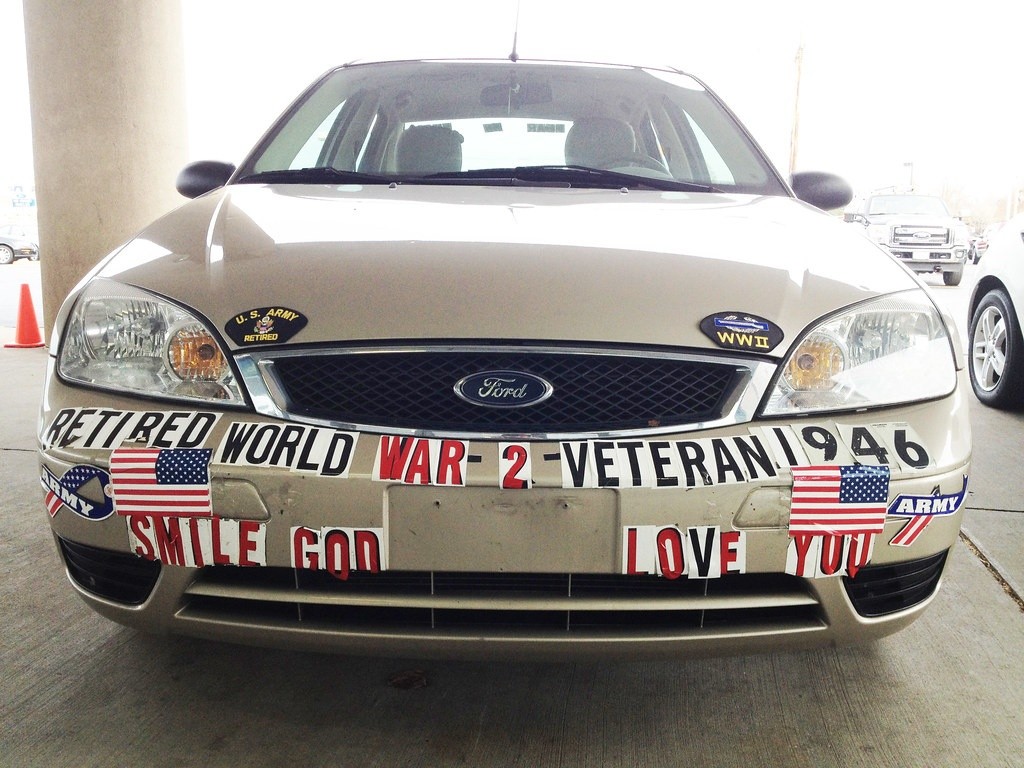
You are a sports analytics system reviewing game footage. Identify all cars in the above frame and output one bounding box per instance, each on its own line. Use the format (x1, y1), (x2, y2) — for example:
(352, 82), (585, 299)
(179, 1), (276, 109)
(0, 223), (39, 265)
(39, 54), (973, 657)
(965, 209), (1024, 408)
(842, 189), (970, 287)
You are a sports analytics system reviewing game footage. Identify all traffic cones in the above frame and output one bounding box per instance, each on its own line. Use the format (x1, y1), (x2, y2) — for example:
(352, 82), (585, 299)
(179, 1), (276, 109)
(4, 284), (44, 349)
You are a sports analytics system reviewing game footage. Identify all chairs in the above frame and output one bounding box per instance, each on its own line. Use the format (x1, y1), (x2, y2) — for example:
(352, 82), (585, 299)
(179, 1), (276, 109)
(391, 126), (463, 175)
(564, 117), (636, 172)
